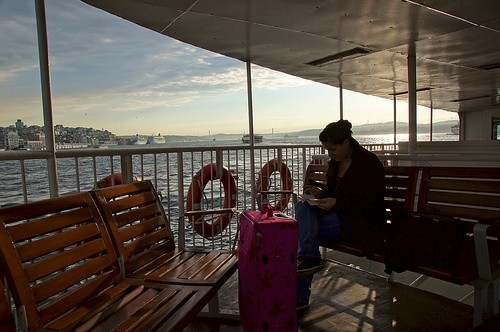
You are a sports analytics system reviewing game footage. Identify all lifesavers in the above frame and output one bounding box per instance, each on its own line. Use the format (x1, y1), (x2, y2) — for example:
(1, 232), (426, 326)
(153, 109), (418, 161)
(82, 172), (159, 276)
(256, 160), (293, 212)
(186, 163), (238, 237)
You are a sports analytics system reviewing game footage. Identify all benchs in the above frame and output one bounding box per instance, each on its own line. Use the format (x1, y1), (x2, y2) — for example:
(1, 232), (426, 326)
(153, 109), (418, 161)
(0, 180), (239, 332)
(303, 141), (500, 327)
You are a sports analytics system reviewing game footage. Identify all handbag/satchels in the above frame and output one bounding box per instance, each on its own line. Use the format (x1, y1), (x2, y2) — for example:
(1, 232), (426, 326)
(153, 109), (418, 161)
(385, 205), (465, 273)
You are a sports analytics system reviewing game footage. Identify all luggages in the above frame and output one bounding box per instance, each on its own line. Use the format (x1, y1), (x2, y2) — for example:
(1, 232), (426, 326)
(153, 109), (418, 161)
(239, 202), (299, 331)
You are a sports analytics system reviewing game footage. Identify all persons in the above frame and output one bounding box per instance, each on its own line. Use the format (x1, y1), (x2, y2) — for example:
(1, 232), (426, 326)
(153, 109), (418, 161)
(293, 120), (389, 312)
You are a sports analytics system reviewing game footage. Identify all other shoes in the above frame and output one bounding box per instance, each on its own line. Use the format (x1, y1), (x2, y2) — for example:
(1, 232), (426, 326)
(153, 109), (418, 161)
(296, 302), (310, 309)
(296, 256), (325, 273)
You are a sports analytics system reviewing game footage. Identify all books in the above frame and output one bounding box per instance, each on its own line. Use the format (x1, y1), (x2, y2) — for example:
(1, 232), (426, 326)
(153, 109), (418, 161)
(300, 192), (322, 207)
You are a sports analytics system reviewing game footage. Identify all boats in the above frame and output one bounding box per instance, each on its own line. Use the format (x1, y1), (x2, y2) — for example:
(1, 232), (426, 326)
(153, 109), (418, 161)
(130, 134), (147, 145)
(80, 157), (93, 165)
(450, 125), (460, 135)
(99, 145), (109, 149)
(241, 134), (263, 143)
(154, 133), (165, 143)
